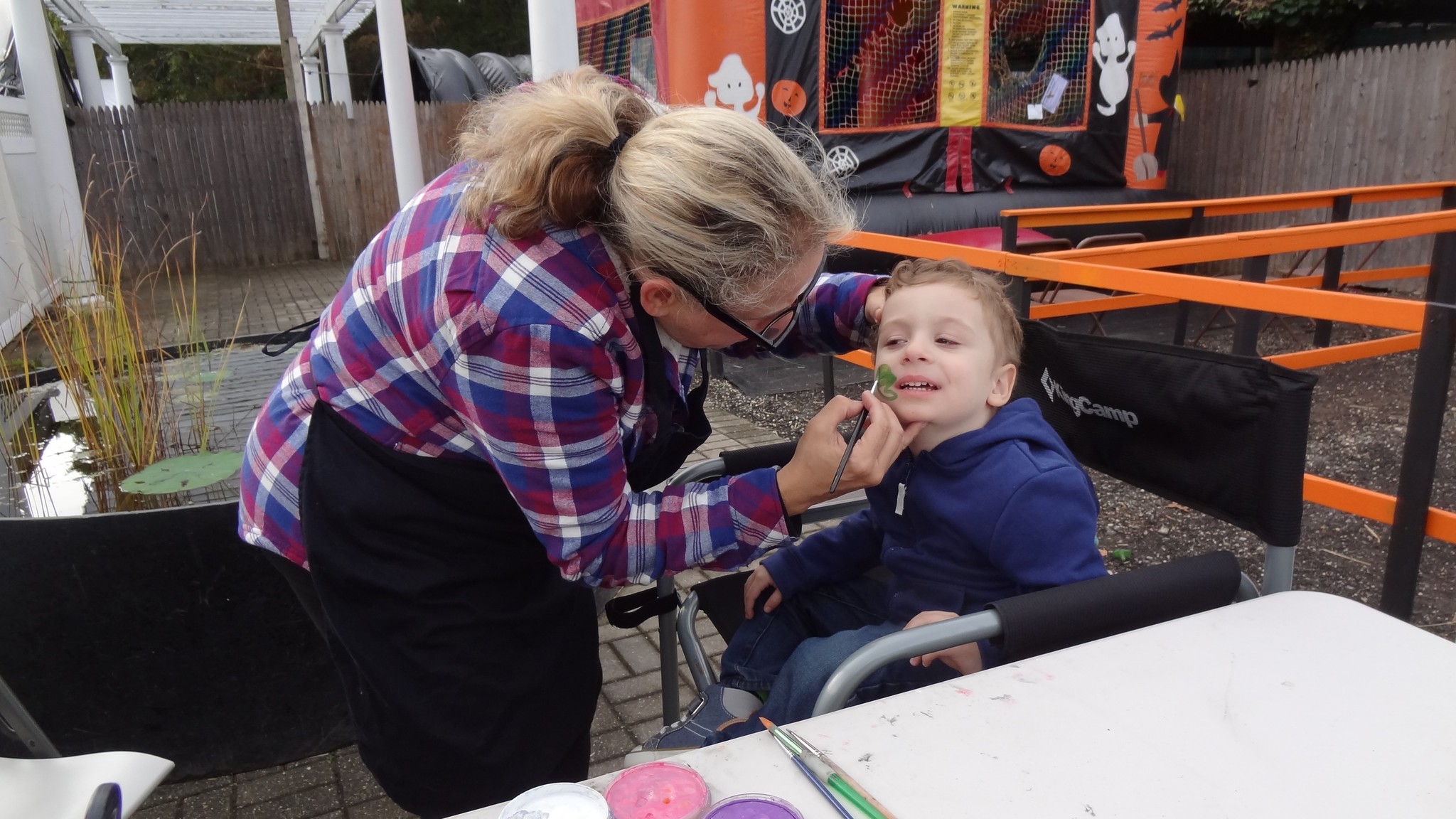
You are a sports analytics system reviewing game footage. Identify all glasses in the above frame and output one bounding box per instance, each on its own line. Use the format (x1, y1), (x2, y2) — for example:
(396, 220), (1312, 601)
(648, 246), (828, 351)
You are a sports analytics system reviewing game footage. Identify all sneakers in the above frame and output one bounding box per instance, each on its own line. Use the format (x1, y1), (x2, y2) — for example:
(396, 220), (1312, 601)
(624, 684), (764, 766)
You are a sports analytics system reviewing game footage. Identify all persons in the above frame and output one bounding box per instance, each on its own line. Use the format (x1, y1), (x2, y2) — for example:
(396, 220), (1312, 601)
(624, 262), (1103, 768)
(233, 63), (928, 816)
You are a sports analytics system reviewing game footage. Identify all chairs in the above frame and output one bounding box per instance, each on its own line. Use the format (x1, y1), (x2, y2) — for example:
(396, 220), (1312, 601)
(607, 319), (1316, 725)
(1193, 222), (1327, 357)
(1016, 238), (1072, 324)
(1260, 239), (1385, 348)
(1030, 232), (1145, 337)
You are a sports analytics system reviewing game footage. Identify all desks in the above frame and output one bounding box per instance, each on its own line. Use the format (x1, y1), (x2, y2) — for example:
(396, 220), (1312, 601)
(443, 590), (1456, 819)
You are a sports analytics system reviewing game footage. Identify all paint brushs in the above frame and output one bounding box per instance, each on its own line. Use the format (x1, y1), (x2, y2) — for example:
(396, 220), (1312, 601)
(758, 716), (897, 819)
(829, 369), (880, 493)
